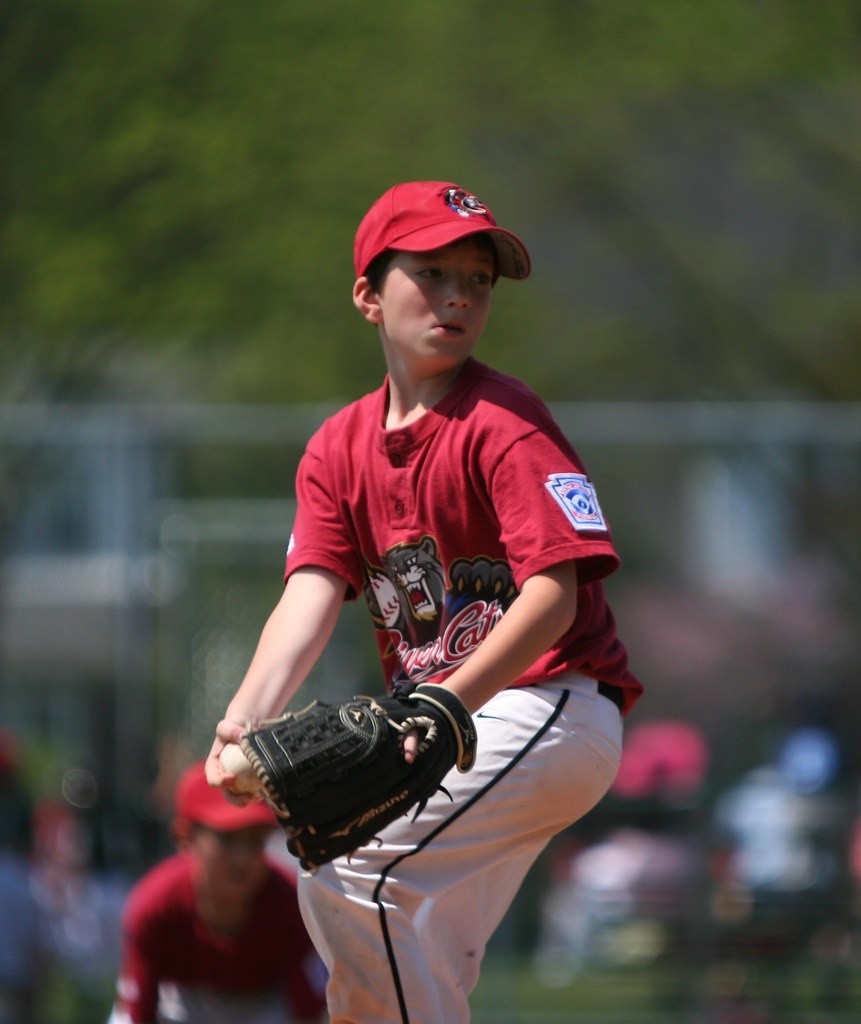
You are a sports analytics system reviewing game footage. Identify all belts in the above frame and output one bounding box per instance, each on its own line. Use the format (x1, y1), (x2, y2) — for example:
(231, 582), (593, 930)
(598, 681), (625, 715)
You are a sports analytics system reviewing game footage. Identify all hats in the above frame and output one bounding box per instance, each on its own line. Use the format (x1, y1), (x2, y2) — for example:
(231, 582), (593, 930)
(354, 181), (531, 279)
(173, 759), (281, 836)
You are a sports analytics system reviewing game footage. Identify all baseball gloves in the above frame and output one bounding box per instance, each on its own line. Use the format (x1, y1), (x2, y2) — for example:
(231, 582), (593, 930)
(238, 677), (478, 880)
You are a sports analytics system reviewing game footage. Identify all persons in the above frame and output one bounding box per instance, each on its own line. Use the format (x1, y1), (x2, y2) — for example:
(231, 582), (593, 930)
(556, 723), (860, 962)
(111, 763), (331, 1024)
(204, 181), (644, 1024)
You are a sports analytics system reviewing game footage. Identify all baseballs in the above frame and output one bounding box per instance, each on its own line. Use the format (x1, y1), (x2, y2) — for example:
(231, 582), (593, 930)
(218, 741), (265, 798)
